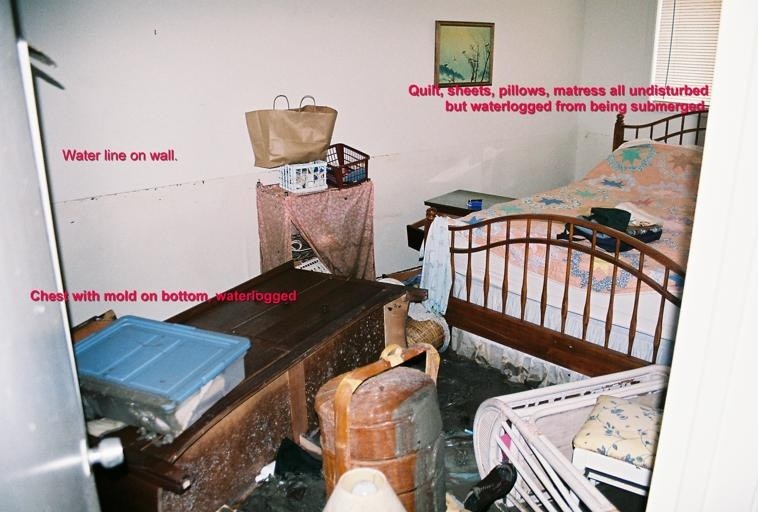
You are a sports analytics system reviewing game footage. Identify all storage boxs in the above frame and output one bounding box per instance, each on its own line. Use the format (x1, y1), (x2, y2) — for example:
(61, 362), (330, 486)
(73, 313), (251, 448)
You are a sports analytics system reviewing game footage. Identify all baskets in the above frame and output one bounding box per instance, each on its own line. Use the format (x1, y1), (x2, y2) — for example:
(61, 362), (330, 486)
(405, 317), (445, 348)
(278, 160), (328, 193)
(326, 143), (369, 190)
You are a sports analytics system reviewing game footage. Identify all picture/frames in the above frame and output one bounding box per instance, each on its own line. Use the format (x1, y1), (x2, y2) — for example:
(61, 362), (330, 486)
(433, 21), (495, 89)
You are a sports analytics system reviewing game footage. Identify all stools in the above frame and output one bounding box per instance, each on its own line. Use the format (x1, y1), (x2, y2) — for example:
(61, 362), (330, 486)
(567, 396), (663, 512)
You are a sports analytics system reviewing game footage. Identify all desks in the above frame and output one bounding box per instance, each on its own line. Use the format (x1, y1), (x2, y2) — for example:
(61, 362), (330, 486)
(425, 189), (515, 217)
(96, 263), (404, 512)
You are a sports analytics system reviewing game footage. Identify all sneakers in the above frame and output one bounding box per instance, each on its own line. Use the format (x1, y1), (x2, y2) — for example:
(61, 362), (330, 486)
(462, 464), (516, 512)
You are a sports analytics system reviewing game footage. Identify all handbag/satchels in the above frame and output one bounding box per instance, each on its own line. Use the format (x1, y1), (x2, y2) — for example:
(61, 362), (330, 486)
(245, 94), (337, 167)
(564, 208), (663, 253)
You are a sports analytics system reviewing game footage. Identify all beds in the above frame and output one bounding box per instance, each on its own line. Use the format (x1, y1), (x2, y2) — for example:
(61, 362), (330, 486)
(423, 102), (710, 379)
